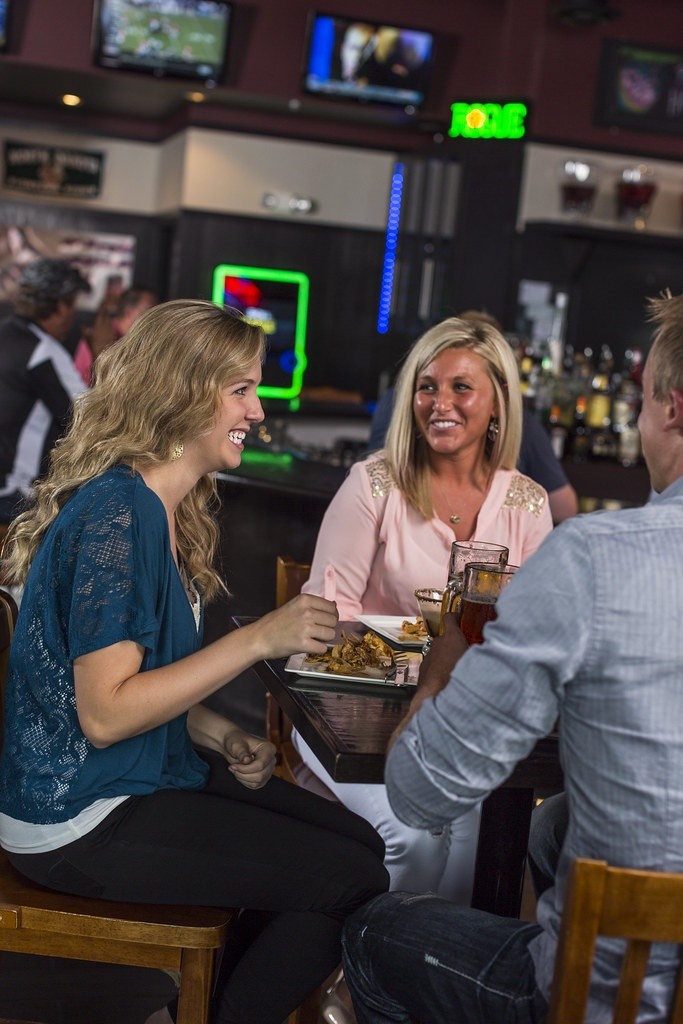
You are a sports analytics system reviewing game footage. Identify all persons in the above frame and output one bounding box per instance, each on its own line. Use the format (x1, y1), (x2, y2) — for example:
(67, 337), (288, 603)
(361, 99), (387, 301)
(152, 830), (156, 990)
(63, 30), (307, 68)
(351, 25), (417, 87)
(293, 309), (580, 921)
(1, 275), (157, 521)
(336, 294), (683, 1024)
(1, 300), (392, 1024)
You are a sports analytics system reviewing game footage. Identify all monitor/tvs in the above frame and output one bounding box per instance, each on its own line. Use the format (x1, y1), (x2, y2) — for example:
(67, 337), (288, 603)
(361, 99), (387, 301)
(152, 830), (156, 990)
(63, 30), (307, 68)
(297, 10), (442, 110)
(91, 0), (240, 81)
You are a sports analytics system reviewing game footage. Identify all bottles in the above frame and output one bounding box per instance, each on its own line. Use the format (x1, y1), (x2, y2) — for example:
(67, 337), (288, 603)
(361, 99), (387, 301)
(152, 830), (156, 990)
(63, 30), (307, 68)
(514, 341), (642, 467)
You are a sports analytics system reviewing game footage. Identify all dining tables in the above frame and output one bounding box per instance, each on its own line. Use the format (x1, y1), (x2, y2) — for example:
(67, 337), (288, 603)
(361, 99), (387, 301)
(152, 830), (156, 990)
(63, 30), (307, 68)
(227, 612), (564, 919)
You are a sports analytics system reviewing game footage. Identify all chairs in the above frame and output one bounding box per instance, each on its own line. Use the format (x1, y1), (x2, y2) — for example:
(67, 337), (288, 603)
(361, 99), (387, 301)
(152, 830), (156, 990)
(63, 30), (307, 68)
(0, 590), (237, 1023)
(267, 554), (311, 780)
(552, 860), (683, 1024)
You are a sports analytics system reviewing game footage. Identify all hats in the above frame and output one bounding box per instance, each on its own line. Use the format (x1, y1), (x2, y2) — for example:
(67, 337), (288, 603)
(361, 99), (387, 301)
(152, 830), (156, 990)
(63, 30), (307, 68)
(19, 259), (91, 299)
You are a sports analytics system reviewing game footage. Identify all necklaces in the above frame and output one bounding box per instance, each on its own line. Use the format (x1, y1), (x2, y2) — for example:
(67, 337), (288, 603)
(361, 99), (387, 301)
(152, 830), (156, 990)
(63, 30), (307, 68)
(432, 474), (479, 523)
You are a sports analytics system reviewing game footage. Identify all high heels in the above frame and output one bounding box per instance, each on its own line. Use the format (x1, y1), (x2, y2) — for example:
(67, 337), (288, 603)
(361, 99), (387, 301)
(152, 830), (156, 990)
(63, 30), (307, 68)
(322, 970), (356, 1024)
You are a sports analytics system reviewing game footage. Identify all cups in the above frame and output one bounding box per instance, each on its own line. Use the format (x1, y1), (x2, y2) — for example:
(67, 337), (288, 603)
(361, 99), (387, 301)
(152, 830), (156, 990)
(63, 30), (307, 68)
(438, 539), (510, 637)
(460, 561), (520, 645)
(415, 587), (445, 637)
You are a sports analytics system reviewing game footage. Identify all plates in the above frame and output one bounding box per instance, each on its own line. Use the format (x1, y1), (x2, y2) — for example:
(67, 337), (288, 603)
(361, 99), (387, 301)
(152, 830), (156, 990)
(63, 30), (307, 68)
(283, 643), (423, 686)
(354, 614), (431, 647)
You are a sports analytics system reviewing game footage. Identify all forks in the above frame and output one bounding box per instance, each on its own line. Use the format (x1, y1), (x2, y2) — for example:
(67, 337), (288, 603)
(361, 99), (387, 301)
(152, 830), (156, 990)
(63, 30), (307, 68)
(384, 650), (407, 683)
